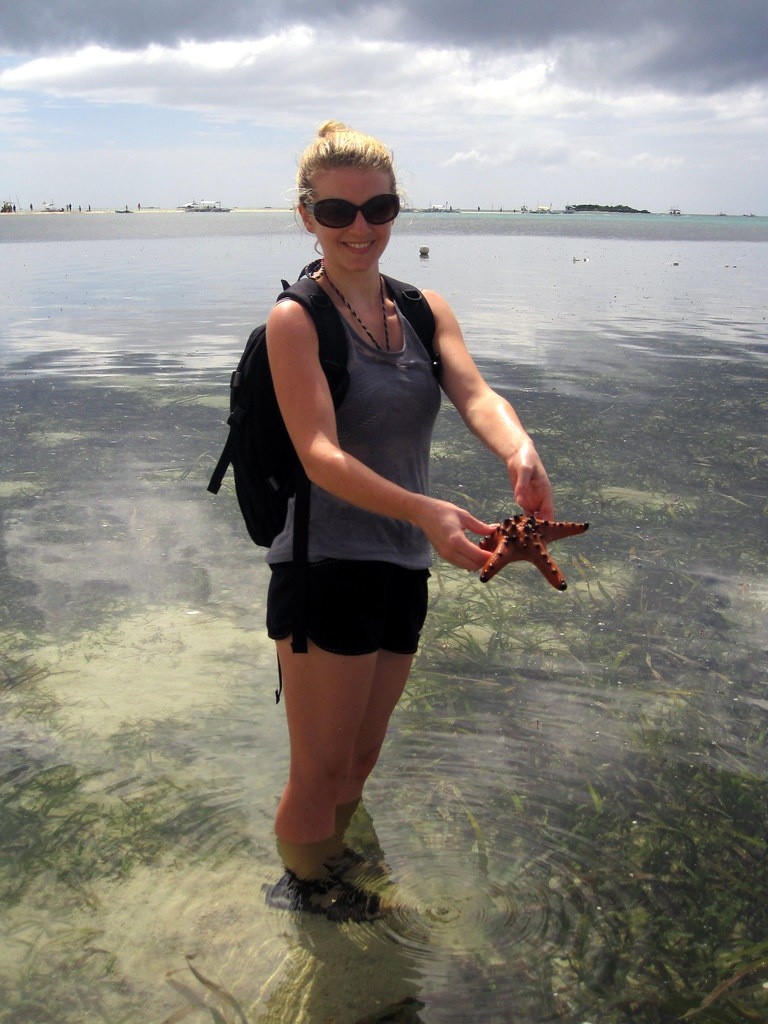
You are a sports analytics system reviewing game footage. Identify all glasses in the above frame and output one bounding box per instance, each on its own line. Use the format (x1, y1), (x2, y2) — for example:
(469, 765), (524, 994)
(306, 194), (402, 230)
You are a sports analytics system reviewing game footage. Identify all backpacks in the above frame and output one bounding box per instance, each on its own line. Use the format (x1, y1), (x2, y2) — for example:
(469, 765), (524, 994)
(206, 273), (443, 548)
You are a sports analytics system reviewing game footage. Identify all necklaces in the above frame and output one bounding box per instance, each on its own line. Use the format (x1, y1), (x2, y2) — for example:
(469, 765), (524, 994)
(322, 266), (392, 352)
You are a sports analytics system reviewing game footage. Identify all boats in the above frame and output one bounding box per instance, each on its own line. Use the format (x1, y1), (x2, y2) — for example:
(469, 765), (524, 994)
(40, 203), (64, 212)
(175, 201), (200, 211)
(561, 204), (576, 215)
(726, 213), (758, 220)
(668, 206), (681, 216)
(716, 212), (727, 216)
(114, 210), (133, 213)
(528, 206), (554, 214)
(520, 205), (529, 214)
(183, 200), (232, 212)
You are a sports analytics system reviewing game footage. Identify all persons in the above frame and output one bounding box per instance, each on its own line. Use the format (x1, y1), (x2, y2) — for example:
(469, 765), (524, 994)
(267, 123), (555, 841)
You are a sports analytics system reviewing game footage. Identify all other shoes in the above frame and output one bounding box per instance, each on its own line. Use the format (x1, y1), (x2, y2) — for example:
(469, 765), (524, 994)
(269, 851), (389, 924)
